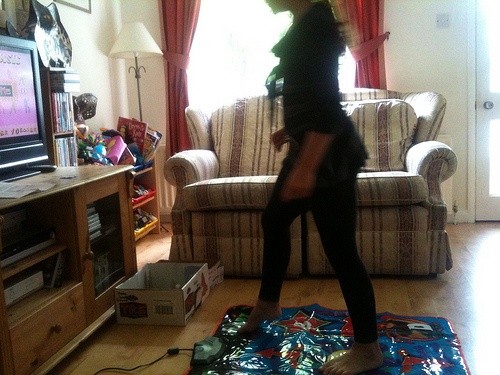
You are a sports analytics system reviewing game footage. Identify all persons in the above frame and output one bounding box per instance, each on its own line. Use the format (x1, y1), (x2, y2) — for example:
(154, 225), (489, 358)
(236, 0), (382, 374)
(119, 124), (128, 143)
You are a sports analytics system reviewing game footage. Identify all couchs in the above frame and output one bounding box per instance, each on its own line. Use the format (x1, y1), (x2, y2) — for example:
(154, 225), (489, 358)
(164, 88), (456, 278)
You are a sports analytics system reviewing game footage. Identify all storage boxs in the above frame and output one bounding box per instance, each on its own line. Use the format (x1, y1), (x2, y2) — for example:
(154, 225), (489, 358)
(116, 263), (211, 325)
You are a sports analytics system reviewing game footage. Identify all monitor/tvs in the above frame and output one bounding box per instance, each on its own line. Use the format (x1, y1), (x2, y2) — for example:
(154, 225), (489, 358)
(0, 35), (48, 182)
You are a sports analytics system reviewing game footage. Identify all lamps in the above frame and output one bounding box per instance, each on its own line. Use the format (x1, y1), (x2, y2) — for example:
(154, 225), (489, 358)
(108, 21), (164, 122)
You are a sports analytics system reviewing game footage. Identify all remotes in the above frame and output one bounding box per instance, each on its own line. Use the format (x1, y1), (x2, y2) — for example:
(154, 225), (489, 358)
(27, 165), (57, 172)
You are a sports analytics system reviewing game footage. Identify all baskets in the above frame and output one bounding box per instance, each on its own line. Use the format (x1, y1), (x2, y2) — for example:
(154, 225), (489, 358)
(73, 93), (98, 122)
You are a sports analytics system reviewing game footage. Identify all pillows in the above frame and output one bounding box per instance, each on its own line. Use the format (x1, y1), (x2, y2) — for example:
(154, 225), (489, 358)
(342, 98), (419, 171)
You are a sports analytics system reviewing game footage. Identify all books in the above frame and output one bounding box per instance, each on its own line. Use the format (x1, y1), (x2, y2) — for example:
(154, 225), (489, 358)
(117, 114), (162, 165)
(51, 91), (77, 166)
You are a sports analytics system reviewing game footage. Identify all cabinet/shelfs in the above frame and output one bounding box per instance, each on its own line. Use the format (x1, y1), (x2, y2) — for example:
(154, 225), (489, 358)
(41, 65), (78, 168)
(0, 164), (138, 375)
(129, 160), (159, 238)
